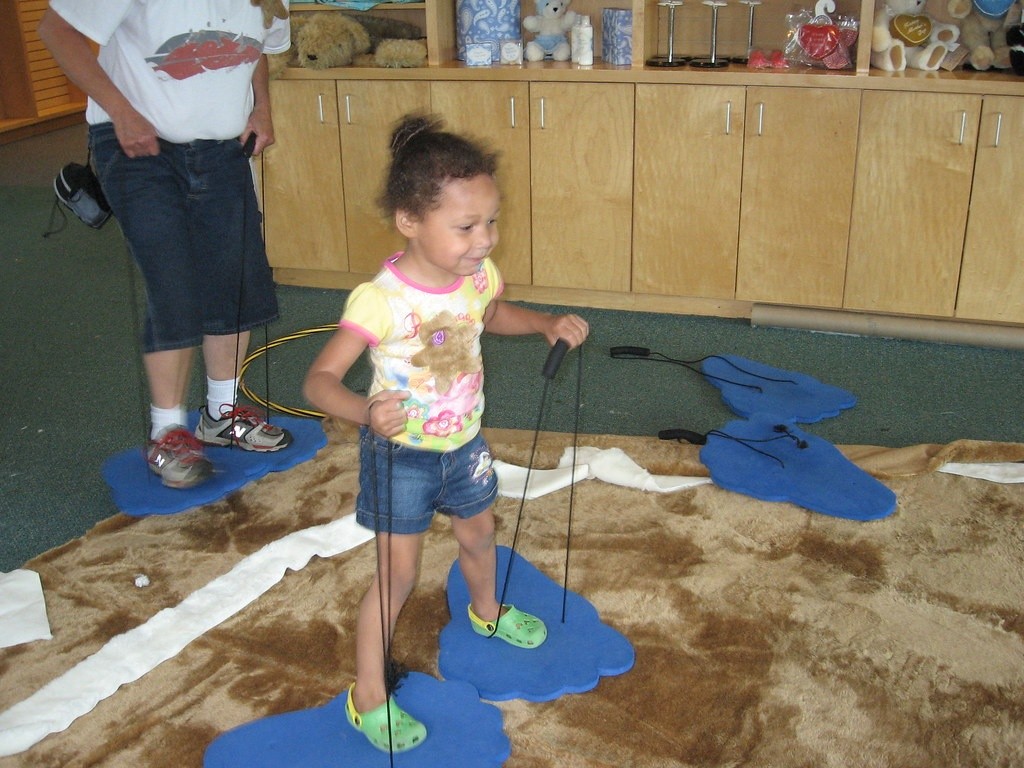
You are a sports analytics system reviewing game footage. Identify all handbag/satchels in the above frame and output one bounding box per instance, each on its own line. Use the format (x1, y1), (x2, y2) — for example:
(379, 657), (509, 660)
(54, 162), (112, 229)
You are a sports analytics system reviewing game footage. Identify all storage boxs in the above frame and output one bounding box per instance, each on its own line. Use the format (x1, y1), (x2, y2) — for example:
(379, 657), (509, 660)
(601, 7), (633, 66)
(499, 39), (524, 66)
(465, 43), (492, 67)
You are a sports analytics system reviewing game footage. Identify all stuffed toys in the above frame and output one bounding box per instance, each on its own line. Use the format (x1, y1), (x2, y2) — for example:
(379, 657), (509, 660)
(871, 0), (960, 71)
(523, 0), (575, 62)
(266, 12), (428, 82)
(948, 0), (1024, 70)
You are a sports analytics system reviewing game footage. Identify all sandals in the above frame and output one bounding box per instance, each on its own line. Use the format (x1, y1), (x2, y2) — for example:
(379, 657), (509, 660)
(345, 681), (428, 753)
(468, 602), (548, 649)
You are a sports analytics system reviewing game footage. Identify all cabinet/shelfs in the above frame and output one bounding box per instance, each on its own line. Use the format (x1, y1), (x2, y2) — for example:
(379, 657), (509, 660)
(263, 2), (1022, 330)
(289, 0), (455, 66)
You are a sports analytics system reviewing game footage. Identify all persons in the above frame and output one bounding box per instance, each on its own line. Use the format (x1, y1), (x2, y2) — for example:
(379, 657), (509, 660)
(37, 0), (295, 490)
(303, 107), (589, 754)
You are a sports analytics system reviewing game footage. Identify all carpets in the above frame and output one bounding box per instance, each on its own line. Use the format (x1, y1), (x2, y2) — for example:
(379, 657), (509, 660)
(0, 417), (1024, 767)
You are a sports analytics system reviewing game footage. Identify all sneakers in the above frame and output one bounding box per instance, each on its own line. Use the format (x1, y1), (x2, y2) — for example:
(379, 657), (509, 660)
(193, 404), (293, 453)
(146, 424), (213, 489)
